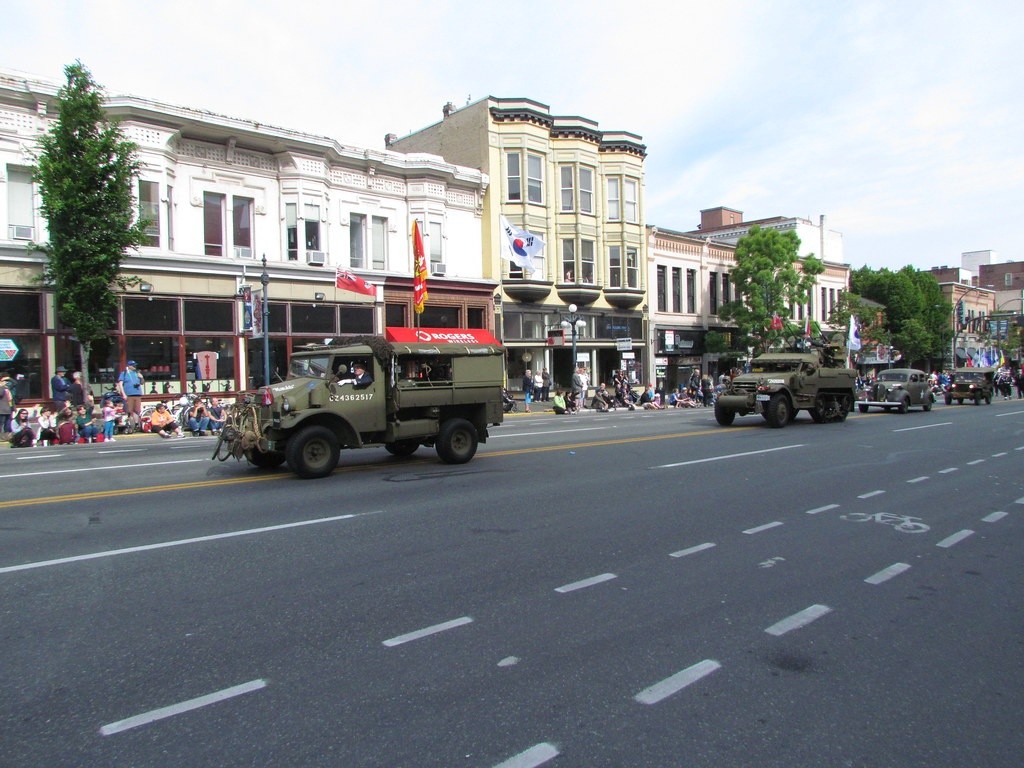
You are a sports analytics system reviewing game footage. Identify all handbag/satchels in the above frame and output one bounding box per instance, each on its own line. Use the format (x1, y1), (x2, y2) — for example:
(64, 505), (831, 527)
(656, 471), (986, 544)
(999, 377), (1004, 386)
(141, 418), (153, 432)
(708, 385), (713, 393)
(262, 387), (273, 405)
(40, 429), (56, 439)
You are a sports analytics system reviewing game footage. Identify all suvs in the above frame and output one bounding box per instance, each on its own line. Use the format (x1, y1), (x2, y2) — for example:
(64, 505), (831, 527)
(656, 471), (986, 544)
(943, 367), (995, 405)
(712, 323), (859, 426)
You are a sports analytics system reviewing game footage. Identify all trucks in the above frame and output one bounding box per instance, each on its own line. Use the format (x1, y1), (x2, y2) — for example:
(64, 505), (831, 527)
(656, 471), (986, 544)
(211, 334), (506, 480)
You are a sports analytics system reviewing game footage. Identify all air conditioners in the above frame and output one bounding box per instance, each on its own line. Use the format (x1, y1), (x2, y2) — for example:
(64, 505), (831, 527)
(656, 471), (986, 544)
(306, 251), (326, 265)
(431, 263), (445, 275)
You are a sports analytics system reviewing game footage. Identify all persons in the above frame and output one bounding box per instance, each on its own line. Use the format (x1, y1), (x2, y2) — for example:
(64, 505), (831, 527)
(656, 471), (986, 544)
(51, 366), (94, 407)
(151, 404), (185, 438)
(8, 373), (25, 399)
(0, 376), (17, 442)
(719, 367), (744, 384)
(188, 397), (227, 437)
(522, 367), (552, 413)
(565, 269), (574, 282)
(11, 400), (122, 448)
(855, 374), (873, 392)
(993, 367), (1024, 400)
(670, 369), (714, 409)
(337, 359), (373, 387)
(927, 370), (951, 396)
(612, 368), (641, 407)
(552, 389), (583, 415)
(640, 384), (666, 410)
(572, 366), (591, 409)
(119, 360), (145, 415)
(591, 383), (622, 411)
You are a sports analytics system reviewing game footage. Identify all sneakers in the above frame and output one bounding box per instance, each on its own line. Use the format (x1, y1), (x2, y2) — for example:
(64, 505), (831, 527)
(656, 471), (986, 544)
(110, 438), (116, 441)
(104, 438), (110, 442)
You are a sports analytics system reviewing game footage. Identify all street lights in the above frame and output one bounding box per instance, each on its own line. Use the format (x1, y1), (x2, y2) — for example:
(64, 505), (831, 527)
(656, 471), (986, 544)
(996, 297), (1024, 360)
(260, 251), (272, 387)
(948, 284), (996, 366)
(560, 304), (586, 375)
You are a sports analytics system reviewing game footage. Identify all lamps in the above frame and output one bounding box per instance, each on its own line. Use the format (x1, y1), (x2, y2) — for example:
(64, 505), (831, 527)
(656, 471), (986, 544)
(139, 282), (150, 291)
(316, 293), (324, 300)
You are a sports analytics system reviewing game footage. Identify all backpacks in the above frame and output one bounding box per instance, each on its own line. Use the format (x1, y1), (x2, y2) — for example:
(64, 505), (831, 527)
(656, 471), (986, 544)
(58, 422), (77, 444)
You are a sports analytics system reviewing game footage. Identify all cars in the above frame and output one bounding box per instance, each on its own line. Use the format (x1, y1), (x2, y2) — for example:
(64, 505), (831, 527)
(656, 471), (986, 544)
(855, 368), (933, 413)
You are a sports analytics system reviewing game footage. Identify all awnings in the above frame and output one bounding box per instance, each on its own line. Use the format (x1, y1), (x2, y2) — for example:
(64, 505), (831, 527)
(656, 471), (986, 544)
(386, 326), (504, 346)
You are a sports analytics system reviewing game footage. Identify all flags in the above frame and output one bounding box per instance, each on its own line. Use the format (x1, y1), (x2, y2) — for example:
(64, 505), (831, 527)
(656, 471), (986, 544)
(499, 214), (546, 275)
(337, 264), (377, 296)
(966, 347), (1006, 368)
(846, 314), (861, 351)
(410, 221), (429, 315)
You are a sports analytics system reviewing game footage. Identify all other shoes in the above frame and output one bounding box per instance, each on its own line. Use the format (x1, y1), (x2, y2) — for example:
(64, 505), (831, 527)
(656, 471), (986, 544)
(213, 430), (221, 435)
(200, 431), (208, 435)
(165, 434), (172, 438)
(194, 431), (199, 437)
(177, 434), (185, 438)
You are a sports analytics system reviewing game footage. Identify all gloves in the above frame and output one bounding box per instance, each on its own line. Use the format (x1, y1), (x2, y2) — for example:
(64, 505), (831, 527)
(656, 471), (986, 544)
(337, 379), (351, 386)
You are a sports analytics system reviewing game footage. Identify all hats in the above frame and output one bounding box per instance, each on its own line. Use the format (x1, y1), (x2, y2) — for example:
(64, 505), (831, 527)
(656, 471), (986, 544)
(352, 358), (366, 366)
(57, 366), (68, 371)
(127, 360), (138, 365)
